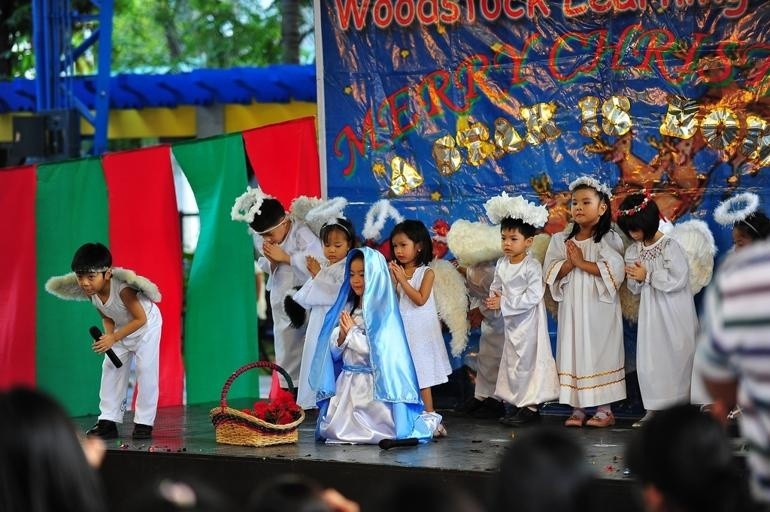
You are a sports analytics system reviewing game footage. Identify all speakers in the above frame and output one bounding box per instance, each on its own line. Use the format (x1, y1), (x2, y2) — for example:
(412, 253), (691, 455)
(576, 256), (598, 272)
(12, 115), (81, 162)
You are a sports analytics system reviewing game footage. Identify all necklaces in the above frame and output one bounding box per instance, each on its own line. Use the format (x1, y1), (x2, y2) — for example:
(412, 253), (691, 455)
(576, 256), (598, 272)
(403, 268), (412, 278)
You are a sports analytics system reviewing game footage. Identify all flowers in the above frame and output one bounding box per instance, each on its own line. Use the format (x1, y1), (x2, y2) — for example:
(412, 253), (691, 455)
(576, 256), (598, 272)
(240, 386), (300, 424)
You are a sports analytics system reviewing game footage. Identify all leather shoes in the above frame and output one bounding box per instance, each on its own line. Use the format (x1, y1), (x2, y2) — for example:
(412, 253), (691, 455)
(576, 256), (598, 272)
(631, 418), (646, 429)
(453, 396), (541, 427)
(564, 407), (587, 427)
(85, 420), (120, 443)
(131, 422), (153, 440)
(584, 407), (616, 428)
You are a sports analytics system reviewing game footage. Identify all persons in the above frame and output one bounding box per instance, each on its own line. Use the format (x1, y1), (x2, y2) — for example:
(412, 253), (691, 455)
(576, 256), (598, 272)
(732, 210), (770, 252)
(696, 241), (770, 511)
(542, 175), (626, 428)
(389, 219), (454, 438)
(71, 243), (163, 440)
(307, 245), (443, 443)
(292, 217), (356, 411)
(473, 215), (561, 427)
(248, 198), (327, 389)
(616, 194), (700, 428)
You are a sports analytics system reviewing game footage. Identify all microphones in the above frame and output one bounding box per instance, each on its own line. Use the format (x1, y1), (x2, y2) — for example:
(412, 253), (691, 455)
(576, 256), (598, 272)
(89, 326), (123, 369)
(379, 438), (418, 450)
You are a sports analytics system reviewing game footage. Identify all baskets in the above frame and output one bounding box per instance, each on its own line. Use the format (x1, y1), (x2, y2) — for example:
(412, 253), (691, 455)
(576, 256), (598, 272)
(209, 360), (306, 449)
